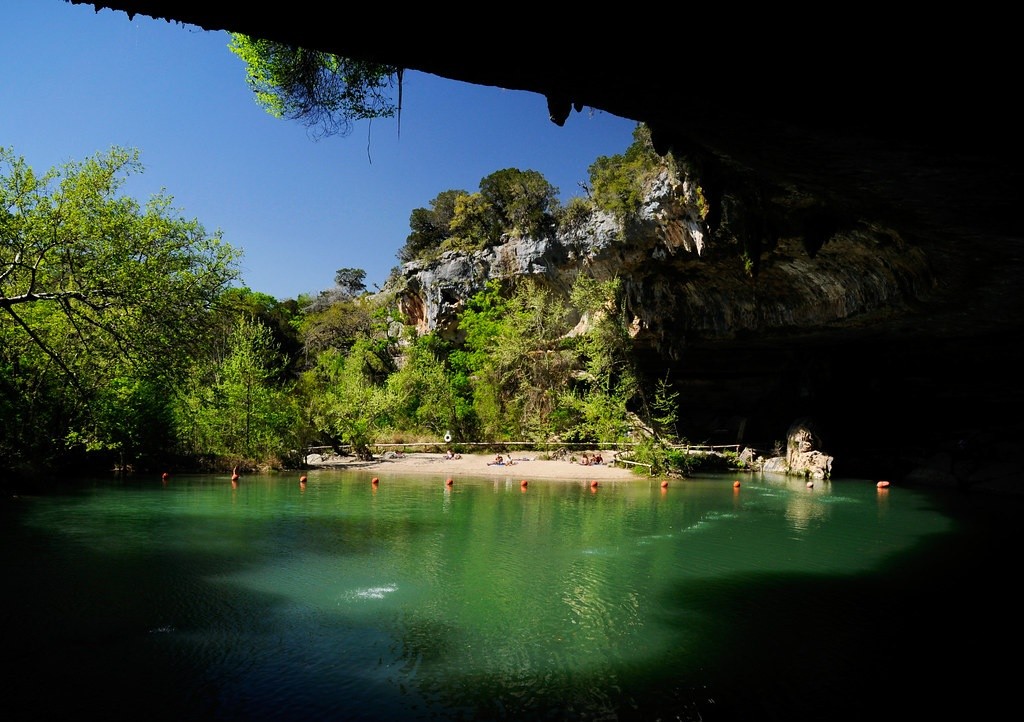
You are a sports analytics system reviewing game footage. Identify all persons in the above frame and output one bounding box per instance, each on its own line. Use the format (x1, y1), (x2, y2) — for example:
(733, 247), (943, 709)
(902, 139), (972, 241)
(579, 452), (603, 465)
(446, 448), (461, 460)
(487, 453), (512, 466)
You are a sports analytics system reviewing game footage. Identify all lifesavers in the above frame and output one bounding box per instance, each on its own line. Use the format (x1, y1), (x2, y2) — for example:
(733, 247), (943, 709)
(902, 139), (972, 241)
(444, 434), (452, 442)
